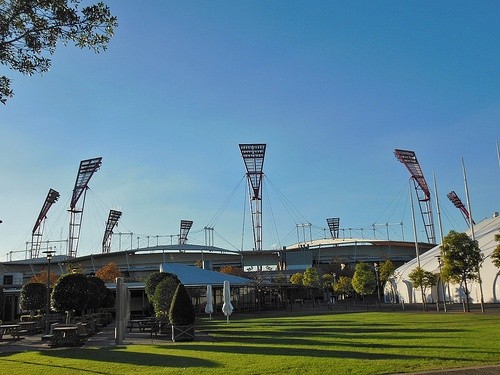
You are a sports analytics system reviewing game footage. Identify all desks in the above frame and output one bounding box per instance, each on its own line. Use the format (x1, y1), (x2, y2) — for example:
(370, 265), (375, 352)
(51, 327), (79, 348)
(129, 319), (151, 334)
(142, 317), (158, 331)
(17, 322), (36, 336)
(0, 324), (20, 340)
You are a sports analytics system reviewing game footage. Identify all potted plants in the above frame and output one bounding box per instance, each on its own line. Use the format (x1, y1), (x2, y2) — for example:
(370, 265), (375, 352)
(20, 272), (112, 338)
(145, 272), (196, 343)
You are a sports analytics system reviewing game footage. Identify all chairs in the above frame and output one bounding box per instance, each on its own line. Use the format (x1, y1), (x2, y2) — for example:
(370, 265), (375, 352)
(127, 325), (157, 333)
(0, 326), (37, 341)
(49, 334), (87, 347)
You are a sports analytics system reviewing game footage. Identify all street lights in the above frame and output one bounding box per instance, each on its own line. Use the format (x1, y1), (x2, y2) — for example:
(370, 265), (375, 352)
(42, 250), (58, 333)
